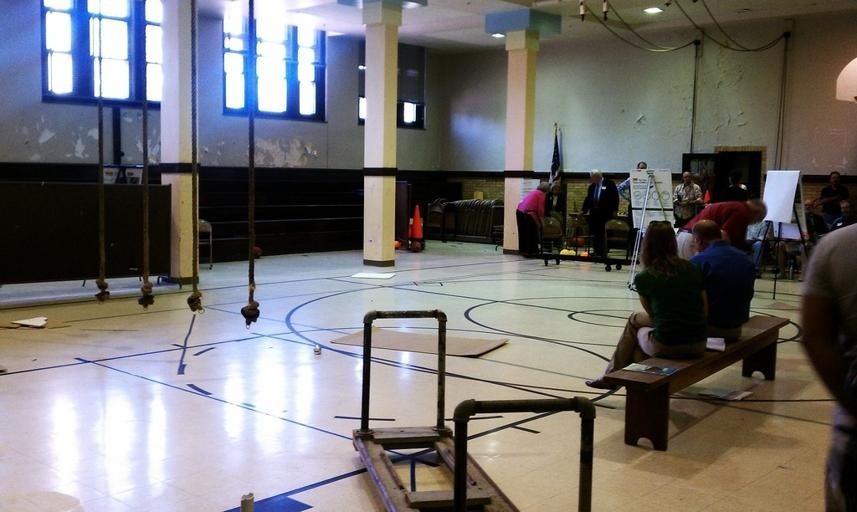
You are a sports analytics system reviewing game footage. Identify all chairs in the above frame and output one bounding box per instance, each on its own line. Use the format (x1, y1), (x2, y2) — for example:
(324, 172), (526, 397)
(784, 251), (801, 279)
(540, 218), (563, 253)
(198, 219), (214, 270)
(605, 220), (630, 259)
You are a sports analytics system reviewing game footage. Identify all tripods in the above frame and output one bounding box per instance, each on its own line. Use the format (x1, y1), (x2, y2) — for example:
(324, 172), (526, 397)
(626, 174), (668, 290)
(753, 204), (810, 300)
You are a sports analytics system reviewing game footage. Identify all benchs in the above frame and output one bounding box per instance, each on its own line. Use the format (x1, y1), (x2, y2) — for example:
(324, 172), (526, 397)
(604, 315), (791, 449)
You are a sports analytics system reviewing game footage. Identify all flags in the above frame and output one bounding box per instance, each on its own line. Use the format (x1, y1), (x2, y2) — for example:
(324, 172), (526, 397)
(549, 130), (562, 185)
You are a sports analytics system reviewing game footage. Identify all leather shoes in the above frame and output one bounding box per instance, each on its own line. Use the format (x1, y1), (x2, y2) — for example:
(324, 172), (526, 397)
(586, 379), (617, 390)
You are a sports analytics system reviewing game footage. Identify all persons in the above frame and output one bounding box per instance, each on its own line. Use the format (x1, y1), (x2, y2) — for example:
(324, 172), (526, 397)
(545, 181), (565, 249)
(617, 161), (856, 281)
(799, 222), (855, 510)
(685, 220), (756, 345)
(516, 182), (550, 259)
(578, 167), (619, 256)
(584, 221), (707, 385)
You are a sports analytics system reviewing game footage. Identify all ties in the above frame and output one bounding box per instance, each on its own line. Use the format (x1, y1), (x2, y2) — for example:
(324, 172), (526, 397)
(595, 184), (599, 201)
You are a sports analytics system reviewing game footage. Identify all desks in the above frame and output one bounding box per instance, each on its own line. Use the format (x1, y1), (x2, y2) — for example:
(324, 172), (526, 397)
(569, 213), (628, 250)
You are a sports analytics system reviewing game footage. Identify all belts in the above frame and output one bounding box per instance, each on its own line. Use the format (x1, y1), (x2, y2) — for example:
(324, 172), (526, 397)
(679, 228), (692, 233)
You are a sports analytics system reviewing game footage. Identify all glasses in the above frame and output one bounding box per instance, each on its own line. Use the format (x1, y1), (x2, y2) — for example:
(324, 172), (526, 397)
(650, 221), (671, 226)
